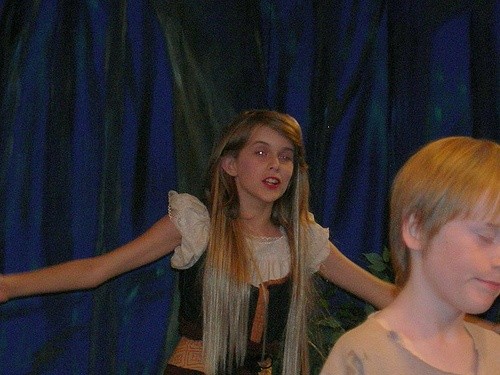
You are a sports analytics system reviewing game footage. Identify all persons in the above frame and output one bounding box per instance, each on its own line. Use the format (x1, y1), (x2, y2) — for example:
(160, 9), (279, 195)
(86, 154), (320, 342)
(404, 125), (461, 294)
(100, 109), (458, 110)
(0, 110), (500, 375)
(319, 136), (500, 375)
